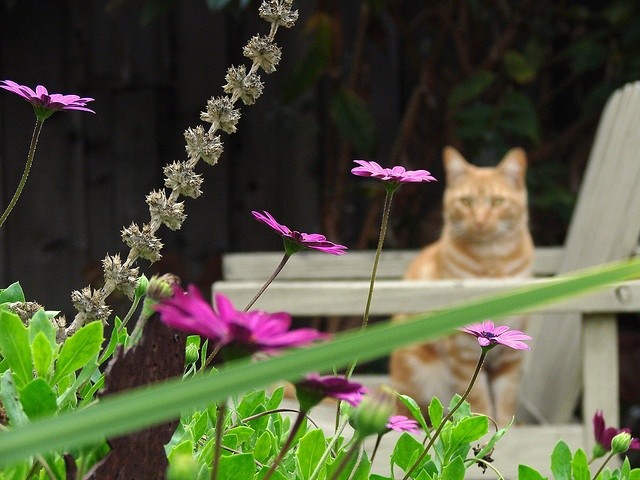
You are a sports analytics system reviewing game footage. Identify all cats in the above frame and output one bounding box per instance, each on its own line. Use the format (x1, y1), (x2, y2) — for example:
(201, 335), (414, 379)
(389, 147), (535, 425)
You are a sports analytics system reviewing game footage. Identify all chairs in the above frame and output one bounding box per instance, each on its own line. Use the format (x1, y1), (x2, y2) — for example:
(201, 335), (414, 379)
(214, 83), (640, 478)
